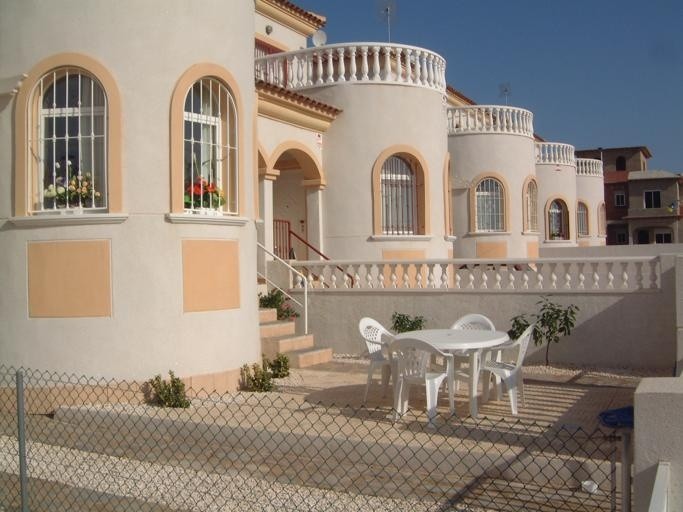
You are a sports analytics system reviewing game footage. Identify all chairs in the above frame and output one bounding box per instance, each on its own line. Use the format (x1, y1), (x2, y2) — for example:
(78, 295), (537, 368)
(358, 313), (535, 430)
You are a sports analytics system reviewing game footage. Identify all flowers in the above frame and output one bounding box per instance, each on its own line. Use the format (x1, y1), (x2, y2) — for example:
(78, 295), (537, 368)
(43, 160), (102, 207)
(184, 176), (227, 209)
